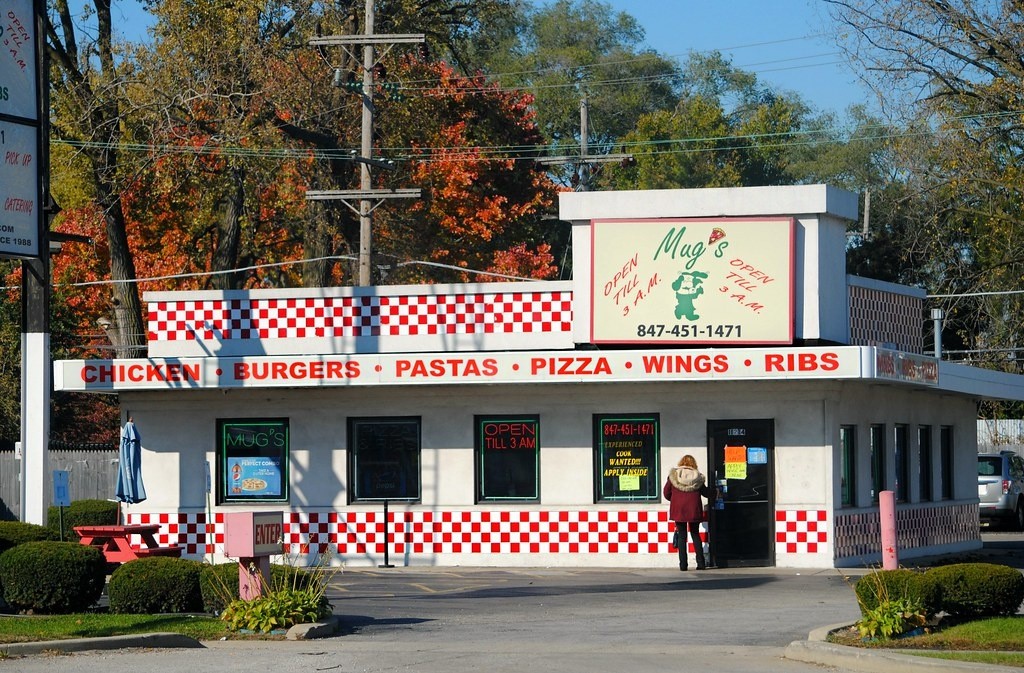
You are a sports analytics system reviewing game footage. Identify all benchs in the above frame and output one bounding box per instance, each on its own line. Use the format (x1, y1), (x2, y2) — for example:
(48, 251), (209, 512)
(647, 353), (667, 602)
(134, 546), (185, 557)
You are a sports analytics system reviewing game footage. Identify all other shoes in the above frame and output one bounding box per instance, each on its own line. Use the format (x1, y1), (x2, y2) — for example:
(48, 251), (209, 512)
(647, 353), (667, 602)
(695, 567), (705, 570)
(680, 568), (687, 571)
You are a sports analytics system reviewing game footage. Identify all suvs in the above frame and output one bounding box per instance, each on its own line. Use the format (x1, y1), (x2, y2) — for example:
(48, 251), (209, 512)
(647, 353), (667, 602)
(978, 450), (1024, 530)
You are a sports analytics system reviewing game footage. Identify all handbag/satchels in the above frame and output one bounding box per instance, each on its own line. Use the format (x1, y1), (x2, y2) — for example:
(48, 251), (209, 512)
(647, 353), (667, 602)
(672, 533), (679, 548)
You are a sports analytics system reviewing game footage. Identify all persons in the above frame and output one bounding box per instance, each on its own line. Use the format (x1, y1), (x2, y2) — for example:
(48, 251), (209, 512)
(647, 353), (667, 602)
(663, 455), (716, 571)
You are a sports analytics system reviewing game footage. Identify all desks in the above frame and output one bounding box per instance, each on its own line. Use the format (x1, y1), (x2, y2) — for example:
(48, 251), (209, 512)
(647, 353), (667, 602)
(73, 525), (159, 561)
